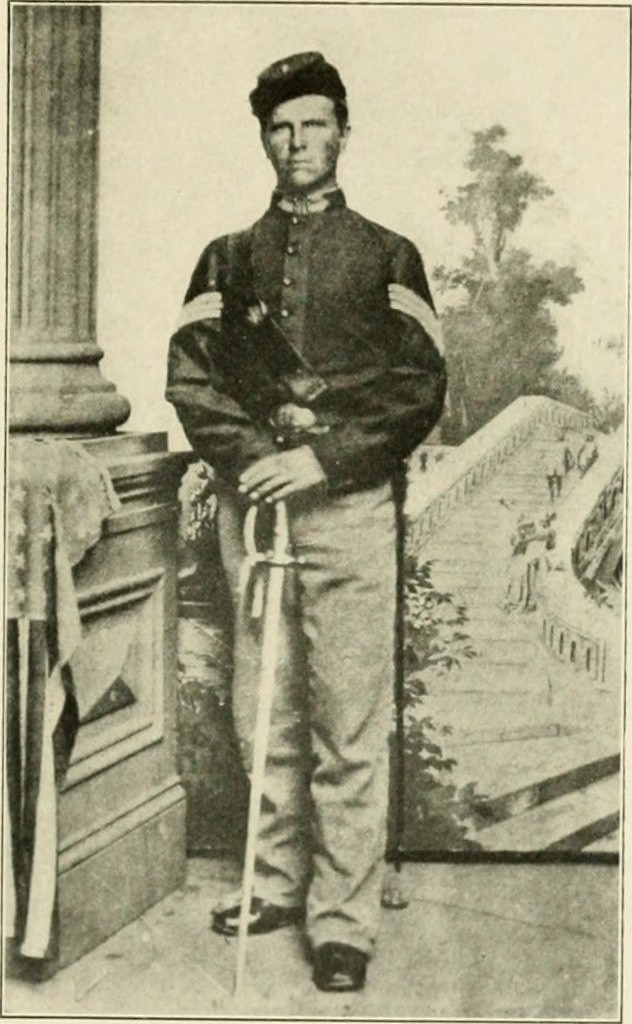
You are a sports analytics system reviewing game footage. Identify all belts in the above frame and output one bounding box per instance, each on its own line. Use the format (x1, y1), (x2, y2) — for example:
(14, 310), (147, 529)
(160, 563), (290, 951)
(271, 424), (326, 448)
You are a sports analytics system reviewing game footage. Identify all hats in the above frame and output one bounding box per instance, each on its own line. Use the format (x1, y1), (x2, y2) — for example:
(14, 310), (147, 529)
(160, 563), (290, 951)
(247, 49), (346, 98)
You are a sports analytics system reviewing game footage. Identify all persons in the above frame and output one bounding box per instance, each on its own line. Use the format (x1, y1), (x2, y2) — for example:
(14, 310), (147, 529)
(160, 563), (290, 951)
(543, 434), (597, 498)
(164, 51), (447, 991)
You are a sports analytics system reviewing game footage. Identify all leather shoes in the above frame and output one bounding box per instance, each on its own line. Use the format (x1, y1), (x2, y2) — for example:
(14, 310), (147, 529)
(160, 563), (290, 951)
(312, 937), (365, 994)
(214, 884), (305, 933)
